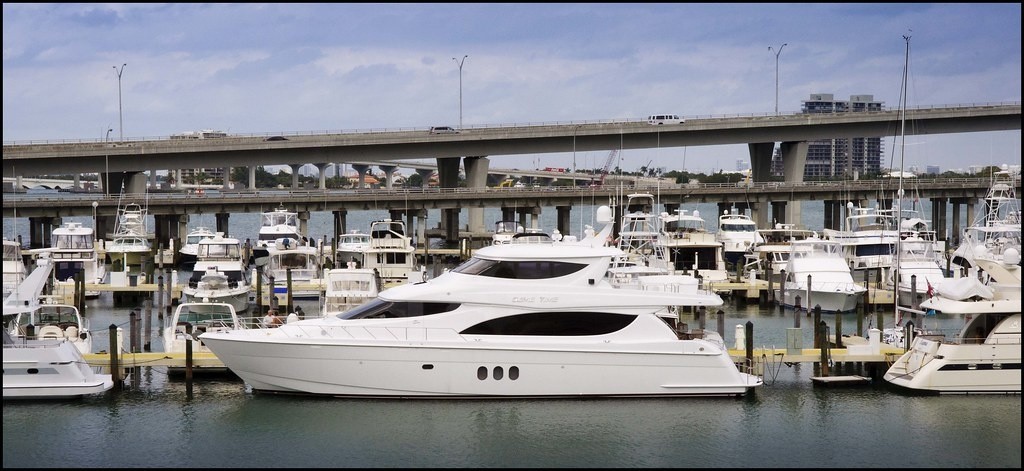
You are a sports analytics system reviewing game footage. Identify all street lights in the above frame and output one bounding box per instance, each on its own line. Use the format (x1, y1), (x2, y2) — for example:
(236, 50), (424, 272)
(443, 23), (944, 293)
(104, 128), (113, 200)
(111, 62), (128, 144)
(768, 42), (788, 119)
(452, 55), (468, 131)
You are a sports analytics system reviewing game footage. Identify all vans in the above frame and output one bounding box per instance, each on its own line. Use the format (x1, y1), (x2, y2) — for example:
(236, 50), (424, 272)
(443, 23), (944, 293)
(514, 183), (526, 188)
(647, 113), (686, 126)
(428, 126), (461, 136)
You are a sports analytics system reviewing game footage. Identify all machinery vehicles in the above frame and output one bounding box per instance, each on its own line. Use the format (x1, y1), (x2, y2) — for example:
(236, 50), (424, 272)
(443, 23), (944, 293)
(588, 149), (618, 190)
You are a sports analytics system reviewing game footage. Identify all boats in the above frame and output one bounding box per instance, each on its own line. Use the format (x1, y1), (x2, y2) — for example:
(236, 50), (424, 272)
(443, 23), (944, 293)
(1, 216), (440, 322)
(607, 165), (1022, 397)
(160, 297), (245, 374)
(6, 303), (93, 356)
(193, 227), (768, 402)
(2, 312), (115, 397)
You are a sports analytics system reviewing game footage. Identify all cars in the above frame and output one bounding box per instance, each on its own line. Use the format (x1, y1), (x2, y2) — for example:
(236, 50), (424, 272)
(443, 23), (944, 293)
(264, 136), (289, 141)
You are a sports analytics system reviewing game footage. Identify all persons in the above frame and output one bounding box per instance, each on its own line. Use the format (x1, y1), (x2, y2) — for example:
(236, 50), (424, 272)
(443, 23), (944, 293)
(263, 310), (273, 329)
